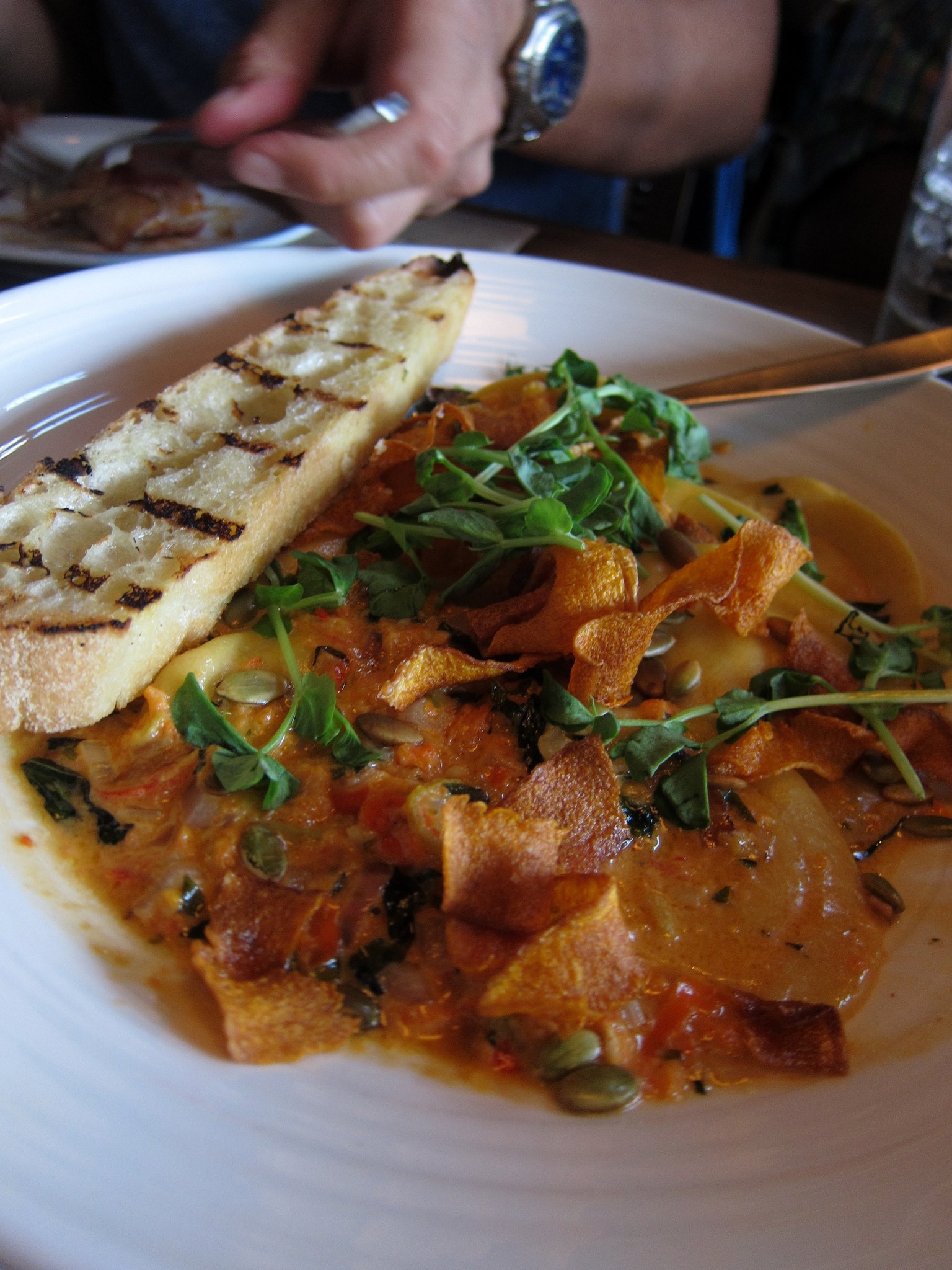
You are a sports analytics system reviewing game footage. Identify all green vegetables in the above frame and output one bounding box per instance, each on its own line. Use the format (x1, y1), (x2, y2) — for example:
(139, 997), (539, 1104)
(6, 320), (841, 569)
(22, 349), (951, 1031)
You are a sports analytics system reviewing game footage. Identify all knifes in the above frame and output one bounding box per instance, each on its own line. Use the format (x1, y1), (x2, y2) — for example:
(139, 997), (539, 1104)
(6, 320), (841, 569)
(657, 329), (952, 405)
(0, 244), (951, 1270)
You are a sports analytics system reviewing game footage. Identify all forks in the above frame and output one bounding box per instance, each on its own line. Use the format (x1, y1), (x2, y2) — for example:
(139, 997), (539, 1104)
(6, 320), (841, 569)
(69, 93), (409, 175)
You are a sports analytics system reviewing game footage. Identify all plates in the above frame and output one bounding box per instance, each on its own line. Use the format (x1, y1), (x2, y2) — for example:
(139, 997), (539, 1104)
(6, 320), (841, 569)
(1, 115), (318, 266)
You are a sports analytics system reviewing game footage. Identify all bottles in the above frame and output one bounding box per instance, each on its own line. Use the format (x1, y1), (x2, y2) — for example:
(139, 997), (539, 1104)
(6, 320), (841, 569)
(876, 130), (951, 385)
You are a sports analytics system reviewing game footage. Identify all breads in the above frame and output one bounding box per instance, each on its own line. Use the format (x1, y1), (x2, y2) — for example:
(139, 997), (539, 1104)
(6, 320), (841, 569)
(1, 251), (478, 731)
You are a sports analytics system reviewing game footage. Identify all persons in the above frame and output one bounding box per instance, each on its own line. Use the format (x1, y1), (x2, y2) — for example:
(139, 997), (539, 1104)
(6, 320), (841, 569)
(0, 1), (780, 251)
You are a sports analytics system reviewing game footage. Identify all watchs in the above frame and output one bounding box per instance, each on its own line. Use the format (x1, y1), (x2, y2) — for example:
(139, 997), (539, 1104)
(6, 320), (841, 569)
(499, 1), (588, 150)
